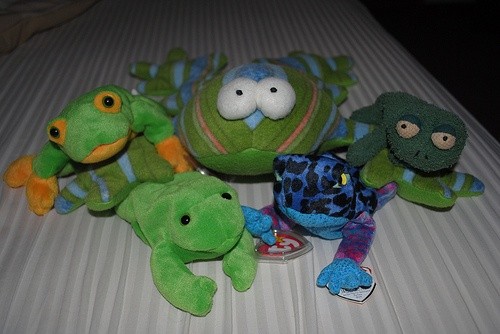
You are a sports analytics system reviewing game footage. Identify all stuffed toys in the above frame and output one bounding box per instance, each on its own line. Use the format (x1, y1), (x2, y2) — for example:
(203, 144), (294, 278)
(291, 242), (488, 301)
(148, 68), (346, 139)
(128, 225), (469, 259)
(4, 83), (200, 217)
(241, 153), (396, 295)
(116, 169), (257, 315)
(345, 90), (467, 174)
(54, 47), (483, 214)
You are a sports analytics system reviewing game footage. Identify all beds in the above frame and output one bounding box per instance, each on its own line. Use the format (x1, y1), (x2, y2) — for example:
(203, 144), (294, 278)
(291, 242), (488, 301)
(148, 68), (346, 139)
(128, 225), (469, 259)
(0, 0), (500, 334)
